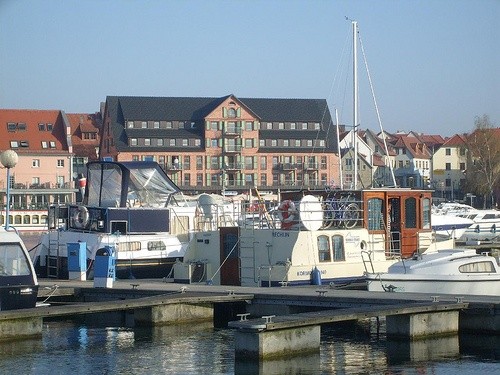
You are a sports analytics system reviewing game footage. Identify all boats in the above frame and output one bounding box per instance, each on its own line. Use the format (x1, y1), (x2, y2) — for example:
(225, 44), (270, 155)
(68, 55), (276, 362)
(34, 19), (499, 297)
(0, 225), (39, 310)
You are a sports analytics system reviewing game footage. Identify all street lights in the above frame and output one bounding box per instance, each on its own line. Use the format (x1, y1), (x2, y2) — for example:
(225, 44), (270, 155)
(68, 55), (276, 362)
(0, 150), (19, 231)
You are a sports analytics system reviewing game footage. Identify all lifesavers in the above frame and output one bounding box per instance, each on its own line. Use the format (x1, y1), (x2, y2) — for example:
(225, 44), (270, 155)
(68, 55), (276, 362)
(75, 205), (88, 227)
(278, 201), (295, 222)
